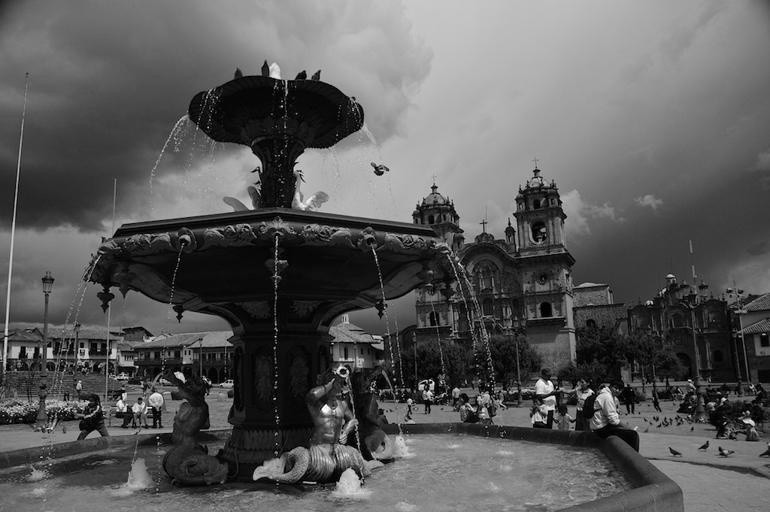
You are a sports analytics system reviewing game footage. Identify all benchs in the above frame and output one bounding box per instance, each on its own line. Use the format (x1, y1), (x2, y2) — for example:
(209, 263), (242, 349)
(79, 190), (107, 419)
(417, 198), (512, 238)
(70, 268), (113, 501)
(108, 406), (162, 428)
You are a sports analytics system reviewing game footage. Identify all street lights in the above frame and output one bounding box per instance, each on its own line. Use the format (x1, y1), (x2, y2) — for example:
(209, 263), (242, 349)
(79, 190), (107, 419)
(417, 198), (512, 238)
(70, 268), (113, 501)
(732, 328), (745, 396)
(511, 314), (527, 408)
(412, 332), (418, 395)
(726, 288), (752, 393)
(671, 265), (709, 422)
(33, 271), (55, 432)
(72, 323), (81, 388)
(199, 338), (202, 377)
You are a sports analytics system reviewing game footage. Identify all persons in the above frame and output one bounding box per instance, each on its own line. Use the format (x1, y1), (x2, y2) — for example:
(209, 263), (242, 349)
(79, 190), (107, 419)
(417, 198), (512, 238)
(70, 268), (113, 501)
(115, 392), (133, 429)
(74, 380), (83, 396)
(422, 383), (434, 416)
(646, 374), (765, 442)
(148, 388), (163, 428)
(407, 397), (414, 414)
(206, 378), (213, 396)
(73, 394), (112, 440)
(141, 379), (150, 397)
(131, 397), (148, 428)
(451, 368), (640, 453)
(308, 362), (360, 446)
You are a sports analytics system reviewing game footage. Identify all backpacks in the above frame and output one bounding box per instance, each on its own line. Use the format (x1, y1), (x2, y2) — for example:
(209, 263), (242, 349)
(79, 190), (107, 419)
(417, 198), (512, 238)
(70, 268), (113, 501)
(583, 393), (602, 419)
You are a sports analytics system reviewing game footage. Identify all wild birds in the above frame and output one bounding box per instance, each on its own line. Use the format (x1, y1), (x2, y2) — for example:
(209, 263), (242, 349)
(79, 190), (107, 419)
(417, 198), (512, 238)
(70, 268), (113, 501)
(699, 440), (711, 452)
(717, 445), (736, 458)
(642, 413), (697, 433)
(233, 66), (242, 80)
(311, 69), (322, 82)
(261, 59), (269, 76)
(369, 161), (392, 178)
(295, 69), (307, 80)
(667, 446), (682, 457)
(758, 448), (770, 456)
